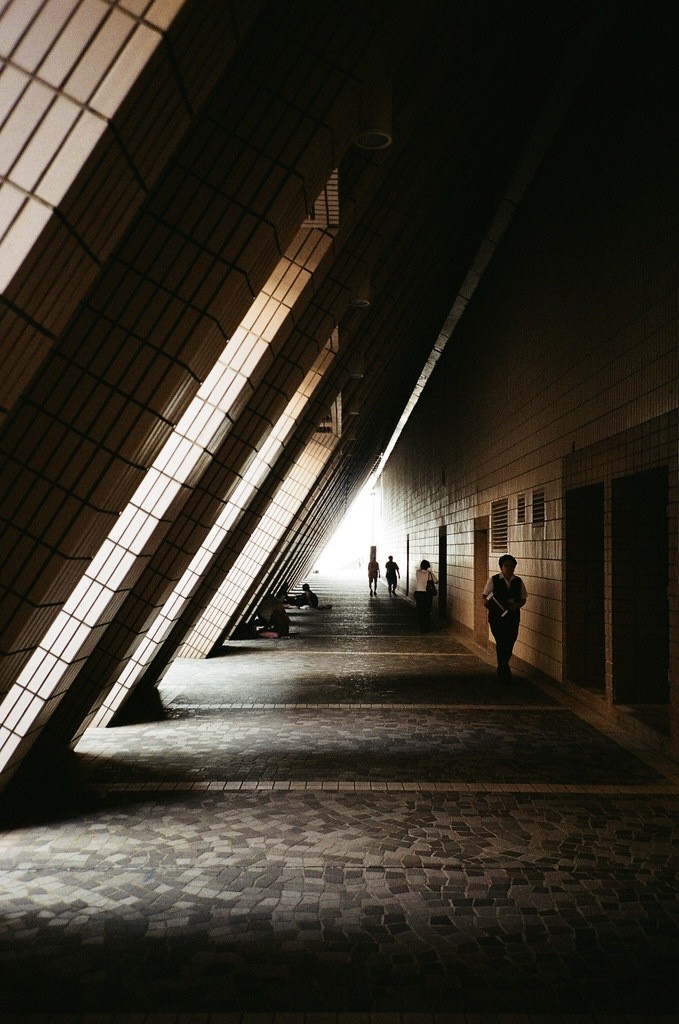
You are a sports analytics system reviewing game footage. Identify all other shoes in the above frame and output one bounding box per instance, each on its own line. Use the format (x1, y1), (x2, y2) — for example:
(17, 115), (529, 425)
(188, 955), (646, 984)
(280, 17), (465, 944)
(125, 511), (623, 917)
(388, 590), (396, 597)
(369, 590), (377, 596)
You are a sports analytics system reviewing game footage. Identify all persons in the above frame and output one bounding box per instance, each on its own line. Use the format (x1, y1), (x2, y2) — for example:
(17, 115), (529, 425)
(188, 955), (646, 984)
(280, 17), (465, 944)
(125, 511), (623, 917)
(296, 583), (318, 608)
(252, 599), (291, 636)
(276, 582), (294, 604)
(385, 556), (401, 597)
(413, 560), (438, 631)
(368, 554), (381, 596)
(482, 553), (529, 682)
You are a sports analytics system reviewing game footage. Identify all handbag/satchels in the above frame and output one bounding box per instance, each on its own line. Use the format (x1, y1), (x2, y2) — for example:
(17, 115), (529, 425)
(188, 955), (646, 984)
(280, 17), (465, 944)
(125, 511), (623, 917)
(426, 572), (437, 596)
